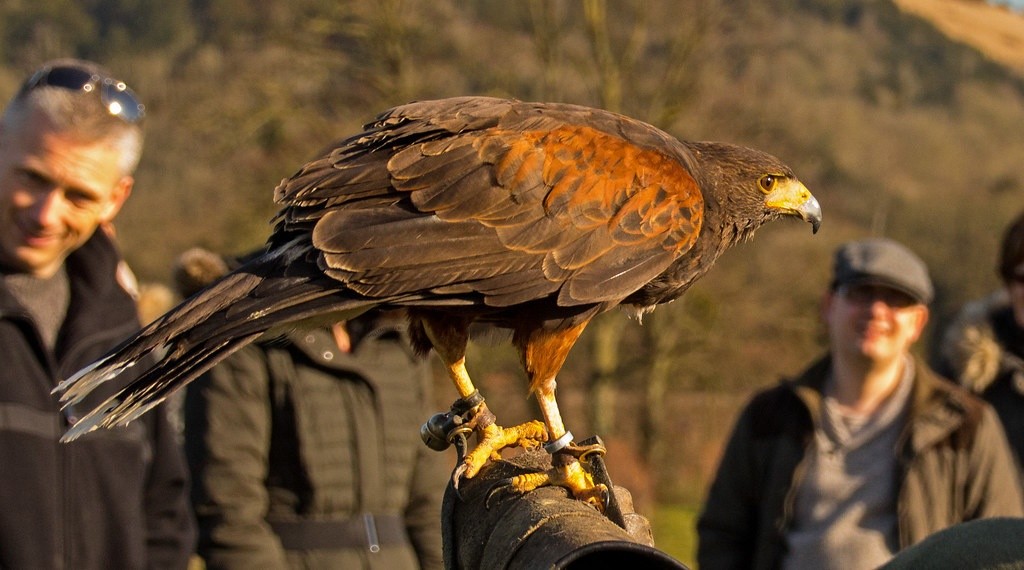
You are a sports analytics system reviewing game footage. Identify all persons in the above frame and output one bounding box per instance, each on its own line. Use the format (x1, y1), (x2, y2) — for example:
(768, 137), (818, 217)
(693, 231), (1024, 570)
(0, 58), (198, 570)
(929, 209), (1024, 481)
(438, 434), (1023, 570)
(160, 242), (461, 570)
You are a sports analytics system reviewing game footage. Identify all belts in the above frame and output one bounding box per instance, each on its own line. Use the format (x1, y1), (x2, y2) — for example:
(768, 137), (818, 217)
(267, 516), (409, 551)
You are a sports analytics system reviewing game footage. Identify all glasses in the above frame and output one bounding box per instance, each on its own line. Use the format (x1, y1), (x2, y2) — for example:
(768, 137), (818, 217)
(11, 62), (148, 124)
(836, 284), (915, 308)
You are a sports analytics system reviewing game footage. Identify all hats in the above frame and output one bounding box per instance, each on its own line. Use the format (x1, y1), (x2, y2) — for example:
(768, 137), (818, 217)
(828, 239), (932, 305)
(996, 213), (1022, 281)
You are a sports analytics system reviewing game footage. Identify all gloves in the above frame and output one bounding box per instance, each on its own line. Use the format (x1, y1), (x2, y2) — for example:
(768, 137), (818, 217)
(443, 450), (693, 570)
